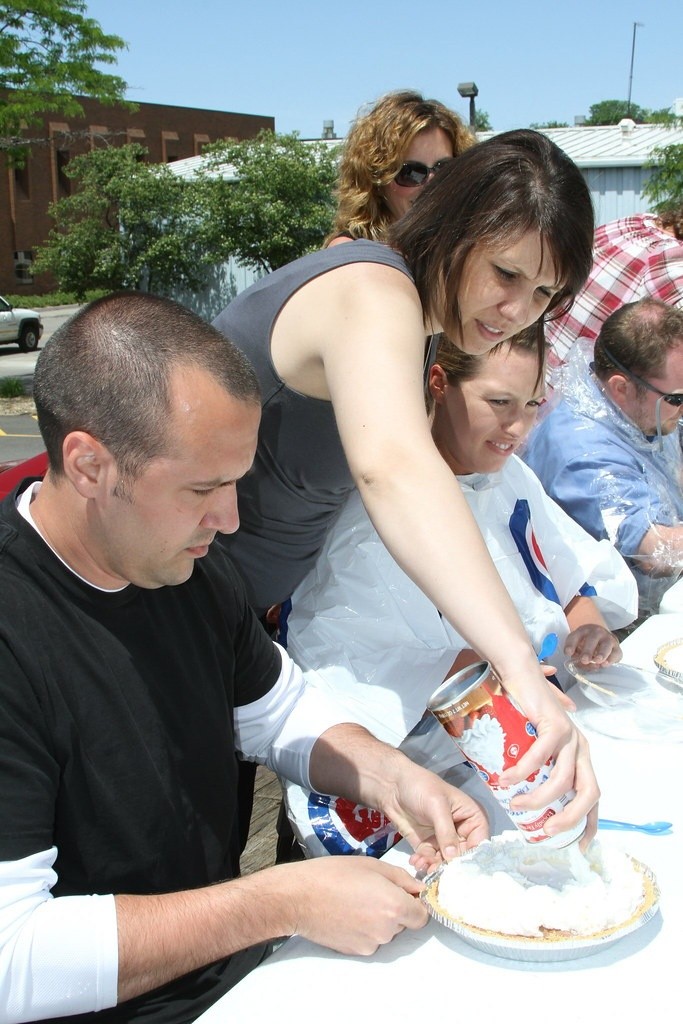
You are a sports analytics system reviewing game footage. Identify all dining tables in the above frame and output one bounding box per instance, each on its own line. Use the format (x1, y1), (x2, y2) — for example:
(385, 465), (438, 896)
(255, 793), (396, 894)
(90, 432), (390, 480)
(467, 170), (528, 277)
(192, 613), (682, 1024)
(659, 577), (683, 614)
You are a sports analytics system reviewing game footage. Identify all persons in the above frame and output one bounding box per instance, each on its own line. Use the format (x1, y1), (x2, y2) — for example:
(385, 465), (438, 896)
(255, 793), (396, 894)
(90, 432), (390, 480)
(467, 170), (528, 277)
(322, 90), (476, 252)
(517, 299), (683, 641)
(205, 129), (601, 876)
(1, 291), (490, 1024)
(536, 200), (683, 427)
(275, 319), (639, 860)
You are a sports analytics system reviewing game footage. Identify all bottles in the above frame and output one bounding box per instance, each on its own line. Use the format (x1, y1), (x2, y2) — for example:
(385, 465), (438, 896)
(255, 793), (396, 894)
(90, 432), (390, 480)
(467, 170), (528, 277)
(427, 660), (589, 853)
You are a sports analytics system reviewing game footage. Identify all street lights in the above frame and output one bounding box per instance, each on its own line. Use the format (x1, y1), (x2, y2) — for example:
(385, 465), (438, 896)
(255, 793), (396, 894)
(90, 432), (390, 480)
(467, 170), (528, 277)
(457, 81), (479, 126)
(627, 22), (646, 116)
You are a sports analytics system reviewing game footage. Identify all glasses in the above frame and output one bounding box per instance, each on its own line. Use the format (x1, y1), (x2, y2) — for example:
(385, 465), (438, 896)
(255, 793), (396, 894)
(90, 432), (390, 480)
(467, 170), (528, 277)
(601, 341), (683, 407)
(387, 158), (454, 188)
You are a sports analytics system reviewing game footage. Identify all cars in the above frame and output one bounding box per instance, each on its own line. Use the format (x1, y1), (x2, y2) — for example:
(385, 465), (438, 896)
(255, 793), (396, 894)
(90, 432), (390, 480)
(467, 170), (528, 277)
(0, 296), (45, 355)
